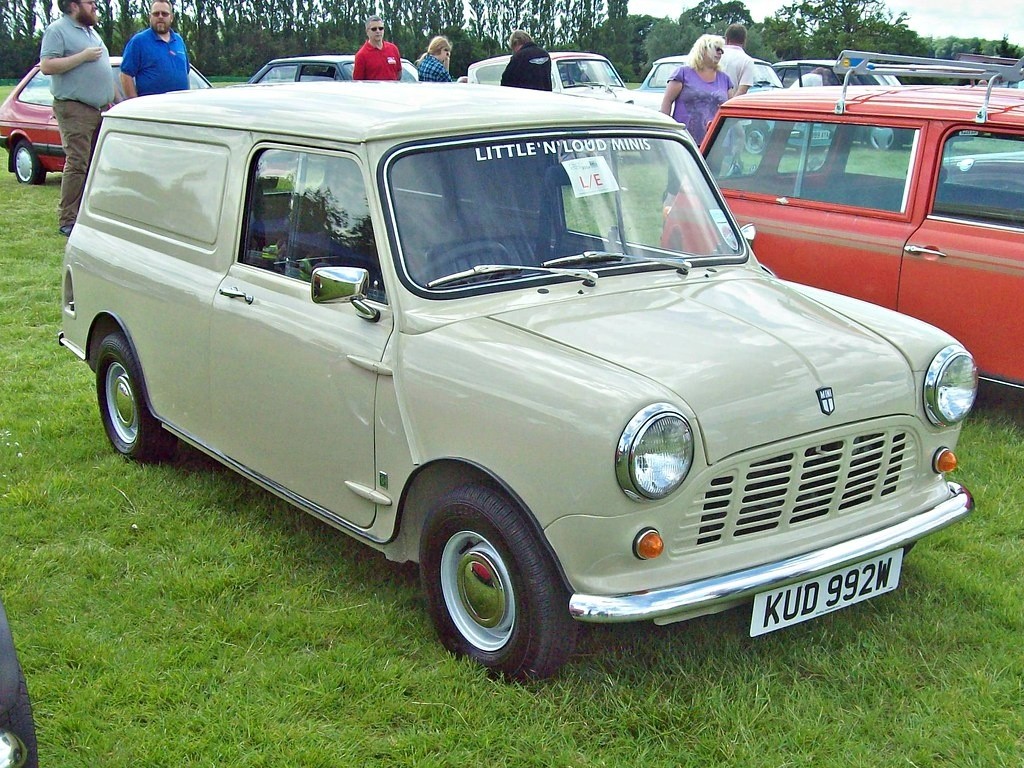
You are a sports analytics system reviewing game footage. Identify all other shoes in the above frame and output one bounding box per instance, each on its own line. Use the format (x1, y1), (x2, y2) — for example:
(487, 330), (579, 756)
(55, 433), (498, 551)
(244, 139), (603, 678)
(59, 226), (71, 237)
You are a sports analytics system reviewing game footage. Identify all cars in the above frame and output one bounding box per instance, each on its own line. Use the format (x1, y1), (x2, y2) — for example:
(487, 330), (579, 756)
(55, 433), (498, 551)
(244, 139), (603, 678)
(772, 59), (918, 150)
(0, 61), (214, 184)
(244, 55), (419, 83)
(55, 78), (979, 679)
(633, 55), (836, 154)
(660, 78), (1024, 392)
(457, 54), (636, 104)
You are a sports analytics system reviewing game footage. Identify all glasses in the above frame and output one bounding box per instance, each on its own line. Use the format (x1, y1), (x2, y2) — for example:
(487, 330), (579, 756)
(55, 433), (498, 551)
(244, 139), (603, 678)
(76, 1), (96, 5)
(151, 12), (170, 17)
(714, 45), (724, 54)
(368, 27), (384, 31)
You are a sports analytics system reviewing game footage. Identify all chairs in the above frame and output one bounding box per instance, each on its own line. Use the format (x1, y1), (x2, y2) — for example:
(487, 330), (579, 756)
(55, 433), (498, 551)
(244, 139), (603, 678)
(426, 236), (542, 281)
(245, 192), (333, 276)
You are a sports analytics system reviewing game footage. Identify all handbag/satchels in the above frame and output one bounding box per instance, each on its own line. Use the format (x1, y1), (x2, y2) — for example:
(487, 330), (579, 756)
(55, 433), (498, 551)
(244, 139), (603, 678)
(725, 152), (743, 176)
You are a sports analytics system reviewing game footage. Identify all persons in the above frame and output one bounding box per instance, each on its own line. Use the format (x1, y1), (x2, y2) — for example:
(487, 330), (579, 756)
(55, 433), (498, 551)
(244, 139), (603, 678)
(353, 15), (403, 83)
(40, 0), (126, 237)
(417, 35), (468, 84)
(715, 22), (755, 101)
(119, 0), (190, 98)
(660, 34), (734, 204)
(976, 79), (988, 85)
(500, 30), (553, 91)
(811, 67), (833, 88)
